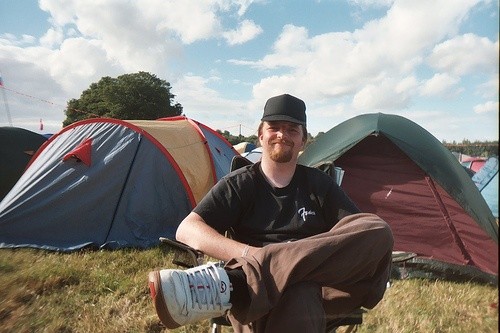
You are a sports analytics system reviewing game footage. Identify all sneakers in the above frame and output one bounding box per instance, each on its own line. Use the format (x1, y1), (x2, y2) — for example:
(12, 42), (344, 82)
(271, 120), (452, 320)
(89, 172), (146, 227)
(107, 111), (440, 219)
(148, 259), (233, 330)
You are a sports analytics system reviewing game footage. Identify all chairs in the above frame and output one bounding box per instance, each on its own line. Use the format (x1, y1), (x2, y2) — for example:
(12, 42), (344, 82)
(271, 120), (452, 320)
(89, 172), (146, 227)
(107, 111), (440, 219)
(158, 156), (417, 333)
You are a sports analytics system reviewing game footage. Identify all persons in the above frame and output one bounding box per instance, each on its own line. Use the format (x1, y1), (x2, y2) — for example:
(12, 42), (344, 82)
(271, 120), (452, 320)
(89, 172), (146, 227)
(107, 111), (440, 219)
(148, 93), (395, 333)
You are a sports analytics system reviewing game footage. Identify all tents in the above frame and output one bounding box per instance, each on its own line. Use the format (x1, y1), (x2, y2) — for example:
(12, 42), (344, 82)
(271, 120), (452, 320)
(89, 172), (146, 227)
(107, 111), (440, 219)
(0, 113), (263, 252)
(296, 111), (500, 283)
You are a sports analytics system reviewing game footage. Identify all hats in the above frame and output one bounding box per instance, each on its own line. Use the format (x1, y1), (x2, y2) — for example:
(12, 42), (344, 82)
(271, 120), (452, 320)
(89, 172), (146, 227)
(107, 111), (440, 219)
(261, 93), (307, 126)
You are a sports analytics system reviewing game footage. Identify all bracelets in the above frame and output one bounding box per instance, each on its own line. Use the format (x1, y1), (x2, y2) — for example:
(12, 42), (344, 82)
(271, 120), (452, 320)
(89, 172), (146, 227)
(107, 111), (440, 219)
(241, 243), (250, 258)
